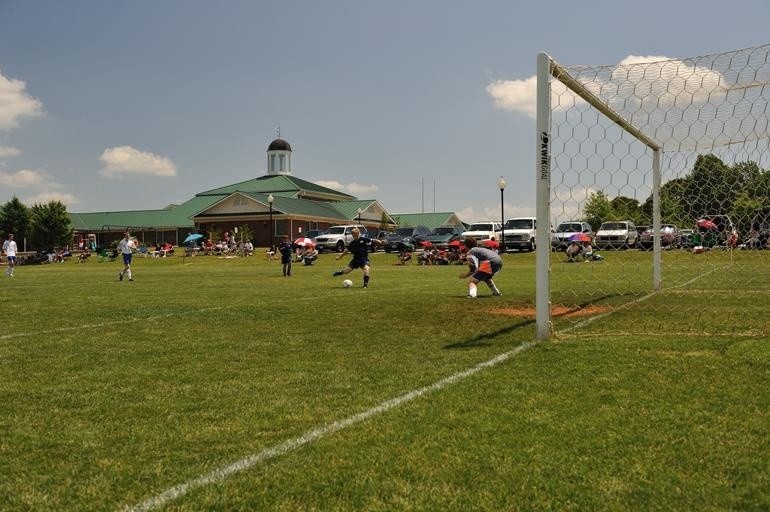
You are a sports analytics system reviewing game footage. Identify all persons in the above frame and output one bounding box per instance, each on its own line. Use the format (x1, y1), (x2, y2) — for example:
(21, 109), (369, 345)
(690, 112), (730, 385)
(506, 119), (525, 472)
(566, 242), (581, 261)
(332, 228), (383, 288)
(115, 231), (136, 283)
(581, 242), (595, 263)
(398, 245), (499, 266)
(723, 222), (738, 253)
(457, 238), (502, 299)
(296, 247), (316, 264)
(265, 243), (277, 260)
(2, 233), (19, 279)
(745, 226), (760, 253)
(190, 230), (254, 259)
(53, 240), (173, 263)
(278, 235), (293, 277)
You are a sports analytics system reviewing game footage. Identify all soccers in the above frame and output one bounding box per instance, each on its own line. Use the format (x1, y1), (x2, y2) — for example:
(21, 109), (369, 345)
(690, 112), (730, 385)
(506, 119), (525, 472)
(343, 280), (353, 288)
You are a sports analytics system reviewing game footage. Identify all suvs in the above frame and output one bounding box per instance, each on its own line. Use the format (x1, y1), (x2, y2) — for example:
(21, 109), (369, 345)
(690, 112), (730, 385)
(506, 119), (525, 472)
(314, 223), (369, 254)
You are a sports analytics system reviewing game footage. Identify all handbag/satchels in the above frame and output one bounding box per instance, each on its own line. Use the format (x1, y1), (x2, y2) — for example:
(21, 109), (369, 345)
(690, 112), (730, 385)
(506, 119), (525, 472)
(438, 258), (449, 266)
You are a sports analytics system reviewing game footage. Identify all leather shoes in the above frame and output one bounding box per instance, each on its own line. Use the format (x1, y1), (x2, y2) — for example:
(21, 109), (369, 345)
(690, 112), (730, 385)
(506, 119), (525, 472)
(466, 295), (477, 298)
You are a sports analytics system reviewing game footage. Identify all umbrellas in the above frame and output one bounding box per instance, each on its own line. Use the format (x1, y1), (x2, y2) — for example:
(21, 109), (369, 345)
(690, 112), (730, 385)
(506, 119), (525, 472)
(568, 232), (591, 242)
(447, 241), (466, 251)
(182, 233), (204, 243)
(481, 240), (499, 249)
(420, 240), (434, 247)
(294, 238), (318, 248)
(694, 217), (717, 231)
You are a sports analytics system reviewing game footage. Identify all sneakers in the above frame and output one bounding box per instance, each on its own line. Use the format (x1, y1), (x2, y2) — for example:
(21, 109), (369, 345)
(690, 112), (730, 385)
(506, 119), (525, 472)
(284, 273), (286, 276)
(332, 271), (338, 277)
(363, 285), (368, 289)
(287, 272), (290, 276)
(492, 293), (502, 296)
(120, 272), (123, 281)
(129, 279), (134, 281)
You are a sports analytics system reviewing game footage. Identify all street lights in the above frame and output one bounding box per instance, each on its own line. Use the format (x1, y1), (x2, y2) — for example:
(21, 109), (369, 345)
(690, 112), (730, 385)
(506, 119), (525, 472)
(498, 174), (508, 254)
(267, 193), (274, 249)
(357, 207), (362, 224)
